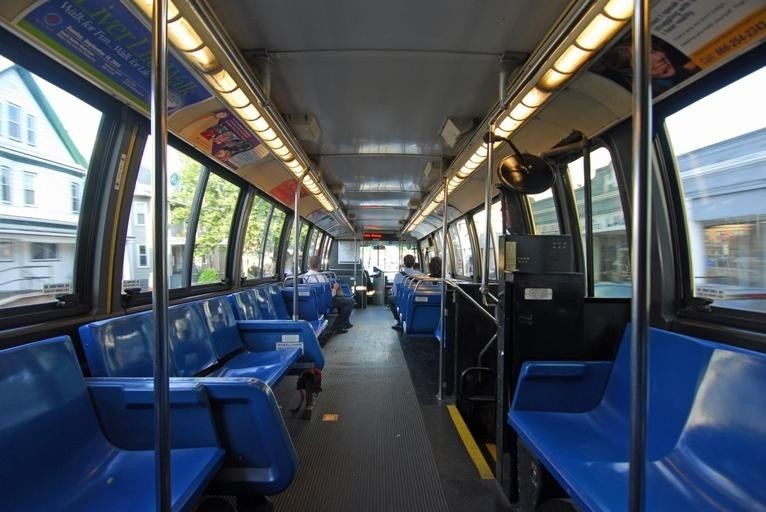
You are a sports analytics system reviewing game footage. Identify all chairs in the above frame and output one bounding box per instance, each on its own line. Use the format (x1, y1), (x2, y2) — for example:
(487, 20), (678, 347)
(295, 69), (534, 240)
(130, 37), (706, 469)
(395, 274), (442, 338)
(283, 271), (352, 328)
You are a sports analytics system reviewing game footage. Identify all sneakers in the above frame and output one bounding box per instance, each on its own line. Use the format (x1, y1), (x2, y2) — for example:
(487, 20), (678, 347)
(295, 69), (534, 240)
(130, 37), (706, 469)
(334, 323), (353, 334)
(392, 324), (401, 330)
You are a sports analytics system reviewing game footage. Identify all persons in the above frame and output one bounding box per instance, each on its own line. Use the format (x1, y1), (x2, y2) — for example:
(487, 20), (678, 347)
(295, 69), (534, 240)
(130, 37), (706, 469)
(388, 255), (420, 330)
(303, 256), (354, 333)
(429, 256), (442, 277)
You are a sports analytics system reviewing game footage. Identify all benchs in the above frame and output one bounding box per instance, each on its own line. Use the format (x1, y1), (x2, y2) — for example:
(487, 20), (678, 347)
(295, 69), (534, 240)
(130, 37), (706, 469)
(0, 284), (329, 512)
(508, 320), (766, 509)
